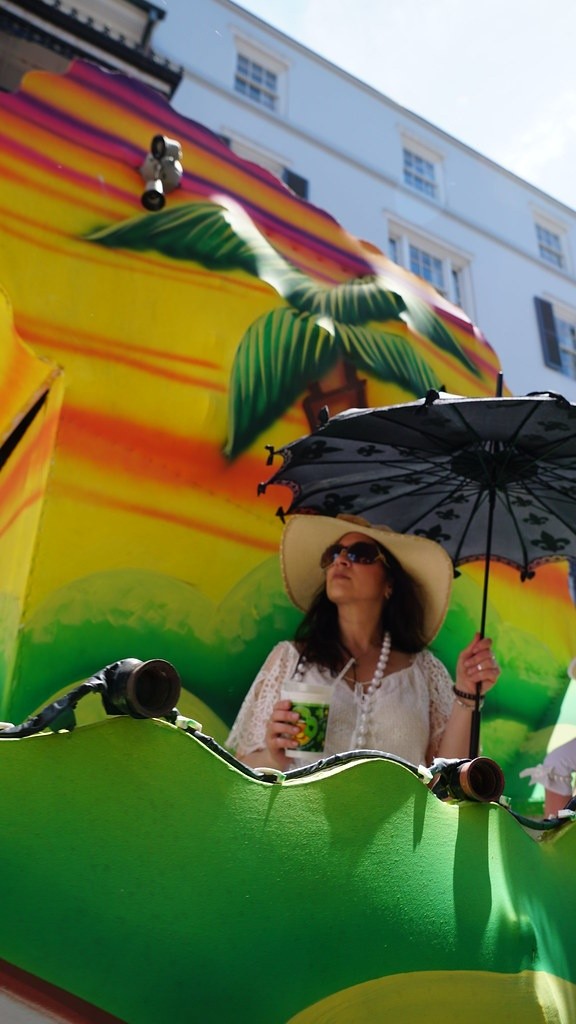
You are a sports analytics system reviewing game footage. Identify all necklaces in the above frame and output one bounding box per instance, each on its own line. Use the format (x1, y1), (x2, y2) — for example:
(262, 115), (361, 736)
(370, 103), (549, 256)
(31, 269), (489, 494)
(292, 630), (389, 750)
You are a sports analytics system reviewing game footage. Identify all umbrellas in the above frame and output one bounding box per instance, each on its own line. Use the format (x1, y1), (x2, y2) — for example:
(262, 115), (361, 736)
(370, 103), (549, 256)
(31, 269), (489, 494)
(258, 373), (575, 762)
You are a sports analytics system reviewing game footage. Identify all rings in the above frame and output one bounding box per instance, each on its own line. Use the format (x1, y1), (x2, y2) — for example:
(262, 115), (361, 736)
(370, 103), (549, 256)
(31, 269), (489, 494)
(477, 664), (482, 671)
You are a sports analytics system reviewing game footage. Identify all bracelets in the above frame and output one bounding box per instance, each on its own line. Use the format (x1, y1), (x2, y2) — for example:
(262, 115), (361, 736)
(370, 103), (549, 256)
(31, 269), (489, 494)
(451, 685), (486, 709)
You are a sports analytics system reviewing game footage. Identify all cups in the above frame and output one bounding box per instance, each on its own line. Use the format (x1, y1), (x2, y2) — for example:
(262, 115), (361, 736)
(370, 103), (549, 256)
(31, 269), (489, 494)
(280, 679), (334, 759)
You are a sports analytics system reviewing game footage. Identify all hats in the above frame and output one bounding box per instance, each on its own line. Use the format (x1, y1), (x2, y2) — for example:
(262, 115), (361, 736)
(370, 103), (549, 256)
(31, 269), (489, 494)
(281, 513), (454, 645)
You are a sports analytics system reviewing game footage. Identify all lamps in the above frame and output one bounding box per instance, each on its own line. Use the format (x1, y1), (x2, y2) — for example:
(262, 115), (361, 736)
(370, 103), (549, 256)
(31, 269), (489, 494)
(89, 649), (201, 722)
(126, 127), (189, 212)
(423, 749), (511, 812)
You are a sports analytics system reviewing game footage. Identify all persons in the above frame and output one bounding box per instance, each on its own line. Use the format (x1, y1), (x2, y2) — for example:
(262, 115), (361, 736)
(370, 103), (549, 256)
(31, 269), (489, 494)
(520, 656), (576, 819)
(224, 513), (500, 784)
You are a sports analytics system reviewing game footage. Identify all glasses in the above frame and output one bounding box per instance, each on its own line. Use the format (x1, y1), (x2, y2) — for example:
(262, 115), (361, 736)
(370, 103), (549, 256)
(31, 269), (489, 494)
(320, 541), (391, 570)
